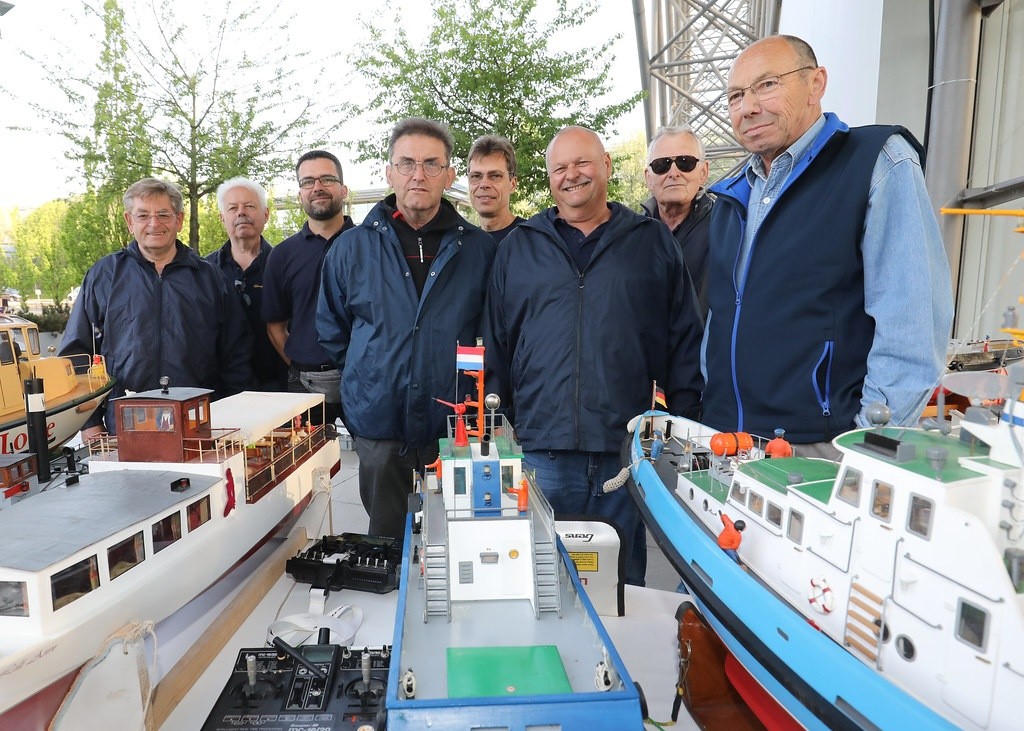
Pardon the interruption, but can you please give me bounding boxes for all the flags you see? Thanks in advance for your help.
[655,386,668,408]
[456,346,485,370]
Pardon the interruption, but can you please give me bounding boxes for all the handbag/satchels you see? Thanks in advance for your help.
[290,369,344,404]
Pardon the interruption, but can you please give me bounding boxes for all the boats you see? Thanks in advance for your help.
[379,337,650,731]
[0,311,119,465]
[0,375,341,731]
[614,386,1024,729]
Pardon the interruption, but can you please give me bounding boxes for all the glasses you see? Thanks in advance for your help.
[647,156,704,176]
[392,159,447,177]
[129,212,179,223]
[299,175,342,189]
[719,66,816,111]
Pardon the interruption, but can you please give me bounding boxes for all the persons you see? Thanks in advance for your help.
[261,150,357,425]
[718,514,746,563]
[765,428,792,459]
[505,480,528,515]
[425,452,442,494]
[57,177,260,447]
[314,118,498,536]
[0,332,22,361]
[651,430,664,467]
[482,125,705,616]
[699,35,955,462]
[304,421,315,434]
[467,135,528,241]
[983,335,991,353]
[641,125,714,295]
[203,176,292,391]
